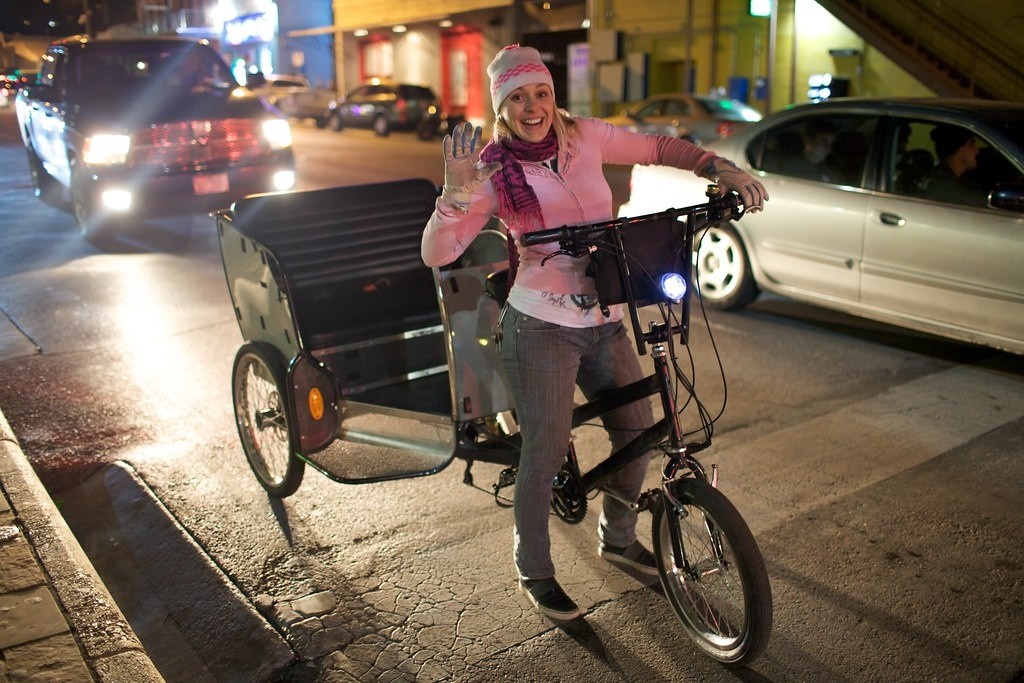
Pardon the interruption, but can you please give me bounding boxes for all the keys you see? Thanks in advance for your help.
[491,324,502,352]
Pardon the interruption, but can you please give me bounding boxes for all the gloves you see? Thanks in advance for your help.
[442,123,502,204]
[701,156,768,213]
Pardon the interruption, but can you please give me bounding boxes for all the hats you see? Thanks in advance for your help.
[487,43,555,116]
[929,125,975,161]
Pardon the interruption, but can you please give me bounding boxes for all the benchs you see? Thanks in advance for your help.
[217,178,478,355]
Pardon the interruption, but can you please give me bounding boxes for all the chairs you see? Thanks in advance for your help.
[898,149,935,198]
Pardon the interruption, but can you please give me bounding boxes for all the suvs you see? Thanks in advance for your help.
[15,35,296,245]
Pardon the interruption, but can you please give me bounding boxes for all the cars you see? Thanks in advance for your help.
[596,92,763,147]
[242,74,331,128]
[329,79,440,137]
[617,97,1024,357]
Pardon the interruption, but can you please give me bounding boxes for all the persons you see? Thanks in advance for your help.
[780,117,844,182]
[866,120,1001,209]
[421,44,769,622]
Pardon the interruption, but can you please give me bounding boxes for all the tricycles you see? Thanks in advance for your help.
[209,177,773,667]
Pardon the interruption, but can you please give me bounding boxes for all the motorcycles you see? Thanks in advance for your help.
[418,104,465,142]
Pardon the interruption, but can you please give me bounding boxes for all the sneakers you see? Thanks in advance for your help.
[598,540,659,576]
[518,576,579,620]
